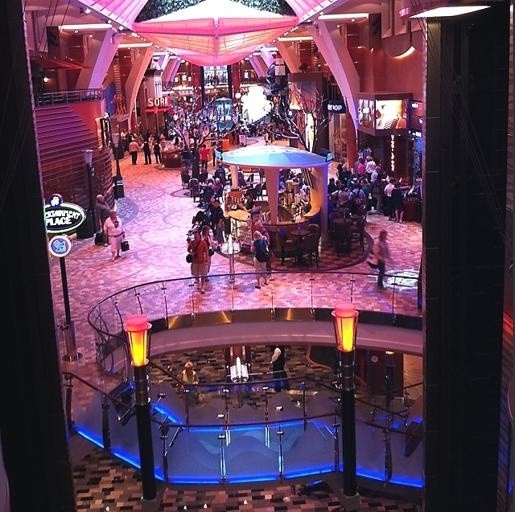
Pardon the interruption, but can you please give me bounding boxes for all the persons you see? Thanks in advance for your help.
[187,230,209,293]
[251,231,270,288]
[123,74,422,267]
[179,361,201,407]
[104,210,125,261]
[96,194,111,246]
[372,229,392,288]
[269,343,291,394]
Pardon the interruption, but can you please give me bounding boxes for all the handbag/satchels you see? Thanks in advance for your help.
[121,241,129,251]
[366,250,379,269]
[186,254,192,262]
[257,249,269,262]
[209,249,214,256]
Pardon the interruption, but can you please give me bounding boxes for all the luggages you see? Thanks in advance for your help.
[95,226,105,245]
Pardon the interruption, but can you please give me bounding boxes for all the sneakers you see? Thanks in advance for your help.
[255,282,268,288]
[111,256,115,262]
[197,276,209,293]
[103,243,108,247]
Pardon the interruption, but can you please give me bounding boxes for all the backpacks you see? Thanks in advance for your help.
[352,190,362,206]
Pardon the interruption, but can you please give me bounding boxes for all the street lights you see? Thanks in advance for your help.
[81,148,100,232]
[121,314,162,510]
[329,300,362,511]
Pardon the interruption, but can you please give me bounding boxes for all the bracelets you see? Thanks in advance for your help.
[270,361,272,365]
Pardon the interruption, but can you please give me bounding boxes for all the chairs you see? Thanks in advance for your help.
[180,171,367,266]
